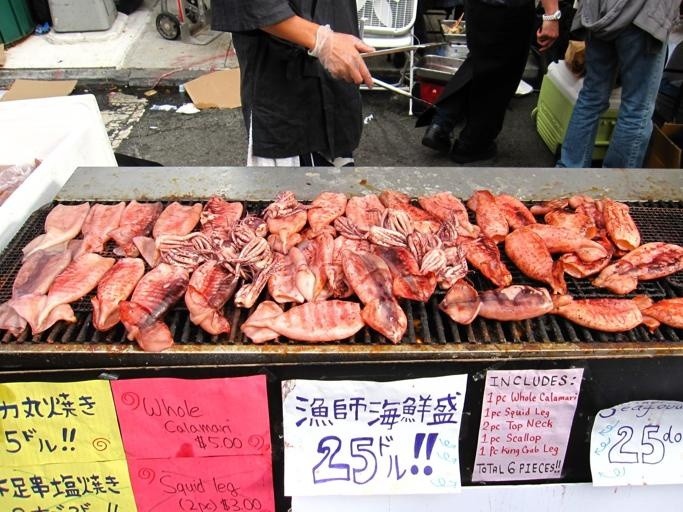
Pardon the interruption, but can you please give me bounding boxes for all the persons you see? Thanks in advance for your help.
[420,0,563,164]
[209,0,375,166]
[556,0,683,169]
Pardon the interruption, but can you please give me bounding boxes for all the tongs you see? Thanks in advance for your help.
[359,41,449,110]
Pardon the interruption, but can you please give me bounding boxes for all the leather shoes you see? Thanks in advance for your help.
[422,123,453,154]
[450,140,497,164]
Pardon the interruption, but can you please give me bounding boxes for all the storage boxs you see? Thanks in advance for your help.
[530,60,622,161]
[651,122,683,170]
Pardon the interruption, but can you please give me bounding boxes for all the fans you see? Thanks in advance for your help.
[356,1,418,37]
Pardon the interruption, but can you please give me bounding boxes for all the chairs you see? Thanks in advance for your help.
[357,29,414,116]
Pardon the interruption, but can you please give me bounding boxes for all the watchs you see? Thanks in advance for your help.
[542,9,562,21]
